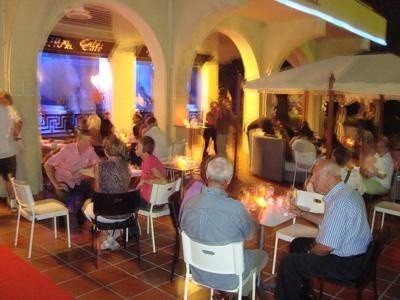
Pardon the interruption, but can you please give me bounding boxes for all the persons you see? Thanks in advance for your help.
[178,154,214,221]
[202,96,397,198]
[42,110,172,252]
[274,158,374,300]
[180,157,268,300]
[0,89,23,214]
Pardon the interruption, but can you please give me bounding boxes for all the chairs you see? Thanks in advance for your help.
[179,230,270,300]
[92,189,143,269]
[292,139,317,187]
[272,190,400,299]
[168,190,181,281]
[138,177,183,253]
[370,200,400,234]
[9,177,72,259]
[158,139,194,187]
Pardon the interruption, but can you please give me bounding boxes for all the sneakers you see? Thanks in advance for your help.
[241,295,250,300]
[214,290,227,299]
[100,240,110,250]
[107,236,120,250]
[10,200,17,209]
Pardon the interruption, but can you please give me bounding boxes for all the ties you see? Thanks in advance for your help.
[344,170,351,181]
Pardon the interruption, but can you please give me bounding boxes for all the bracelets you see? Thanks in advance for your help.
[300,211,304,218]
